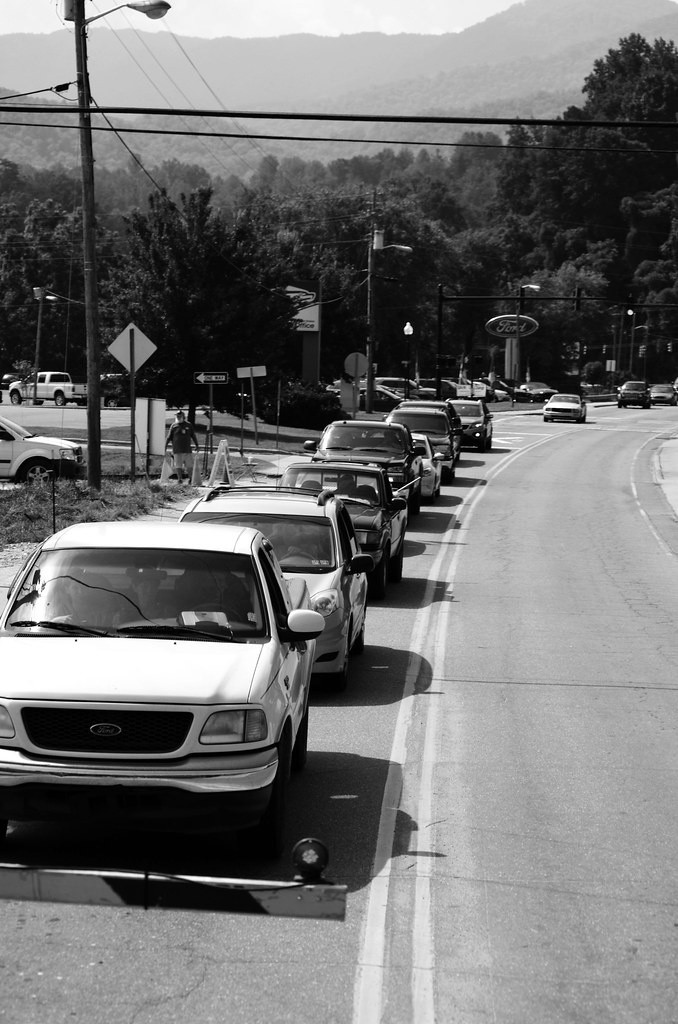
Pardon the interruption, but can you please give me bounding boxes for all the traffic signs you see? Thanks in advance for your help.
[193,371,229,385]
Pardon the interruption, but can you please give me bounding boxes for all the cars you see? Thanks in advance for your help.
[0,415,85,486]
[648,385,678,406]
[410,433,444,506]
[384,401,463,483]
[543,393,587,423]
[326,378,559,412]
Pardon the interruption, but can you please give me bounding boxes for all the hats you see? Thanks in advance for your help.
[175,411,184,417]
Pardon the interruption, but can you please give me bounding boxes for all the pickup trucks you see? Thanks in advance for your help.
[278,461,408,599]
[9,372,87,406]
[303,421,426,523]
[0,520,325,840]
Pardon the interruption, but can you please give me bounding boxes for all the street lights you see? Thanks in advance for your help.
[62,0,171,492]
[366,240,412,413]
[404,320,411,400]
[627,309,648,381]
[33,287,58,402]
[517,285,541,383]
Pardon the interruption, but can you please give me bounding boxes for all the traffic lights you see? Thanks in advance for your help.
[571,285,582,313]
[667,343,673,352]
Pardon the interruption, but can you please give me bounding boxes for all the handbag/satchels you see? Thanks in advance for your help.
[192,454,202,486]
[161,457,174,482]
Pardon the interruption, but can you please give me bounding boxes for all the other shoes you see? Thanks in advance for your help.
[177,479,181,484]
[188,479,192,484]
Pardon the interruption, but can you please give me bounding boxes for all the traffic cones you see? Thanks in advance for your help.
[219,464,229,484]
[190,455,205,487]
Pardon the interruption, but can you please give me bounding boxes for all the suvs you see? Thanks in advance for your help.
[445,398,493,452]
[616,381,651,410]
[179,485,375,696]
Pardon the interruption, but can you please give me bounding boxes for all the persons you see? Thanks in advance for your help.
[376,431,402,450]
[45,570,97,624]
[274,524,320,560]
[121,577,177,625]
[165,409,200,483]
